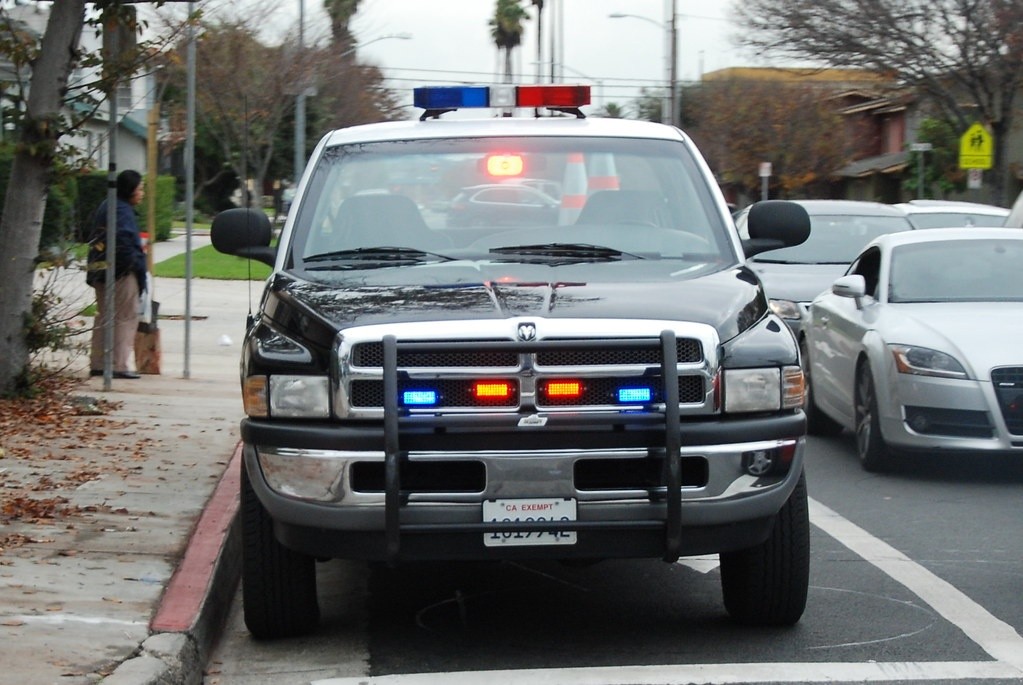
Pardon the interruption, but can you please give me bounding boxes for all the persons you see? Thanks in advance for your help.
[85,170,149,380]
[272,179,290,230]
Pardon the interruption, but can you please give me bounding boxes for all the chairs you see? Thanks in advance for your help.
[327,191,438,252]
[576,189,654,227]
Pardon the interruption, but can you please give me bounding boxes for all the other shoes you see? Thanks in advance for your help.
[90,369,102,375]
[113,371,140,378]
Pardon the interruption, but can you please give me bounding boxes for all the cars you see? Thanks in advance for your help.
[888,188,1023,231]
[794,225,1022,473]
[737,192,917,337]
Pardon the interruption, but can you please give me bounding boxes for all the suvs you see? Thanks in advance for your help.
[442,175,565,229]
[209,83,811,642]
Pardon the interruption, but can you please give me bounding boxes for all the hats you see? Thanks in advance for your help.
[117,170,142,197]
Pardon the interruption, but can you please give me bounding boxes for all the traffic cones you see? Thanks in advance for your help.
[584,152,620,194]
[556,149,588,225]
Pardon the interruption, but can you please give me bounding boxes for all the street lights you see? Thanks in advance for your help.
[291,31,412,190]
[530,58,607,117]
[609,12,681,126]
